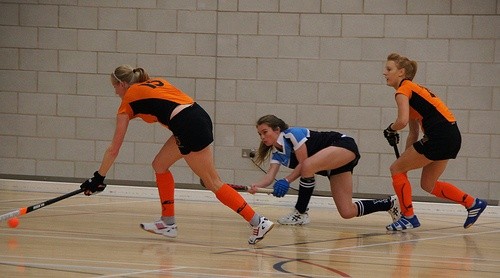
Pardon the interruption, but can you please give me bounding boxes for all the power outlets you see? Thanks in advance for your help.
[241,148,252,158]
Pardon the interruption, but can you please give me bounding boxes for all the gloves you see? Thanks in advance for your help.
[272,178,290,198]
[384,123,399,146]
[81,173,106,197]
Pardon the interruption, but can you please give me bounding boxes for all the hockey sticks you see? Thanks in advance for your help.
[390,132,399,158]
[200,177,275,193]
[0,188,85,222]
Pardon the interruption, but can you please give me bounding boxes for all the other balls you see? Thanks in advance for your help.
[7,217,18,227]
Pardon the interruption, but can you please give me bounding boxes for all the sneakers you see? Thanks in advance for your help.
[386,214,421,231]
[464,197,488,228]
[140,221,178,238]
[277,207,309,224]
[386,194,400,221]
[248,217,275,244]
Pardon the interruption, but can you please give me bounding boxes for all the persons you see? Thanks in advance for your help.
[80,65,275,245]
[246,115,403,226]
[382,54,488,232]
